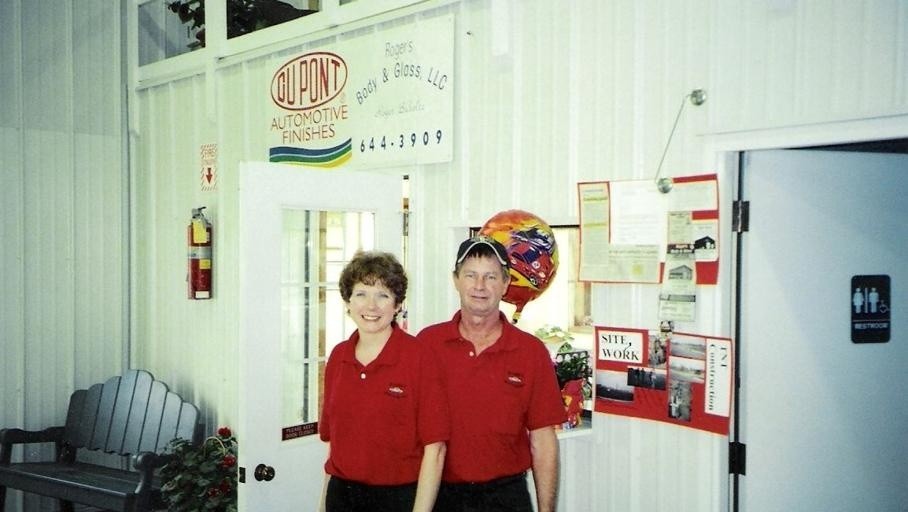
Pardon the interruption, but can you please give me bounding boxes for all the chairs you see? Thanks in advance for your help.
[0,370,200,512]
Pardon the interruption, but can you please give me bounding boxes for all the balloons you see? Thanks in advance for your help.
[476,209,561,323]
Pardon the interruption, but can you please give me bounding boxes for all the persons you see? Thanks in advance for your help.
[417,235,569,512]
[649,340,666,363]
[317,249,452,511]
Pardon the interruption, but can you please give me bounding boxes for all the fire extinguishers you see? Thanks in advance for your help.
[187,206,212,300]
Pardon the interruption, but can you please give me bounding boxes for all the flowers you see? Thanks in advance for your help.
[160,427,238,512]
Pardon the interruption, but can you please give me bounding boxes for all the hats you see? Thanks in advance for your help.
[456,236,511,269]
